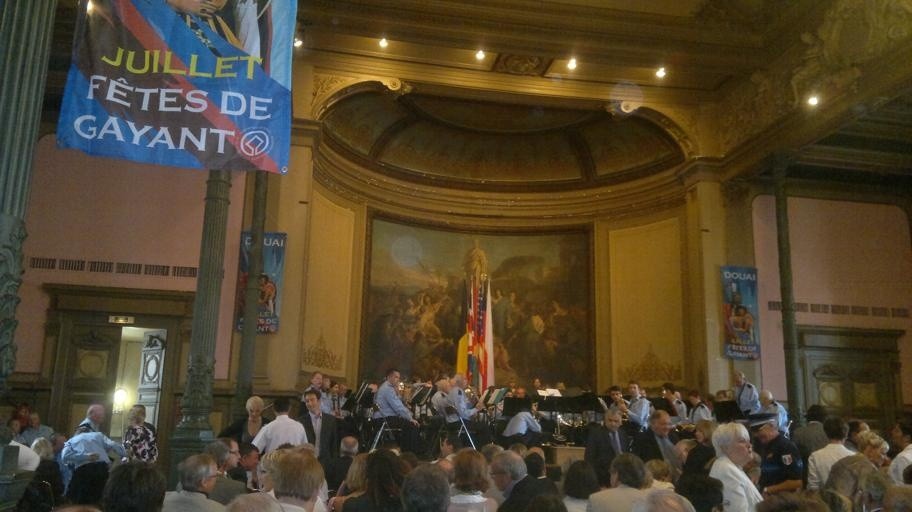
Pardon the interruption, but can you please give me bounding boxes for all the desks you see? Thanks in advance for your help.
[547,444,586,466]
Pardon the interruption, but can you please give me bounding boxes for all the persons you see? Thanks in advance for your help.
[729,305,753,344]
[1,366,912,511]
[88,0,246,153]
[388,289,584,384]
[724,291,742,337]
[260,276,276,316]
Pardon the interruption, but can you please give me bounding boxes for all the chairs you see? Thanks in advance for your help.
[30,480,54,508]
[368,404,403,452]
[434,406,479,450]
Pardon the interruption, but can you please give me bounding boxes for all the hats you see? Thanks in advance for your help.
[746,412,779,430]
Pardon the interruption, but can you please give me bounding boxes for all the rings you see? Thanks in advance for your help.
[200,9,205,14]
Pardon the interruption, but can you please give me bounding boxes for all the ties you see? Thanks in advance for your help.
[611,431,619,455]
